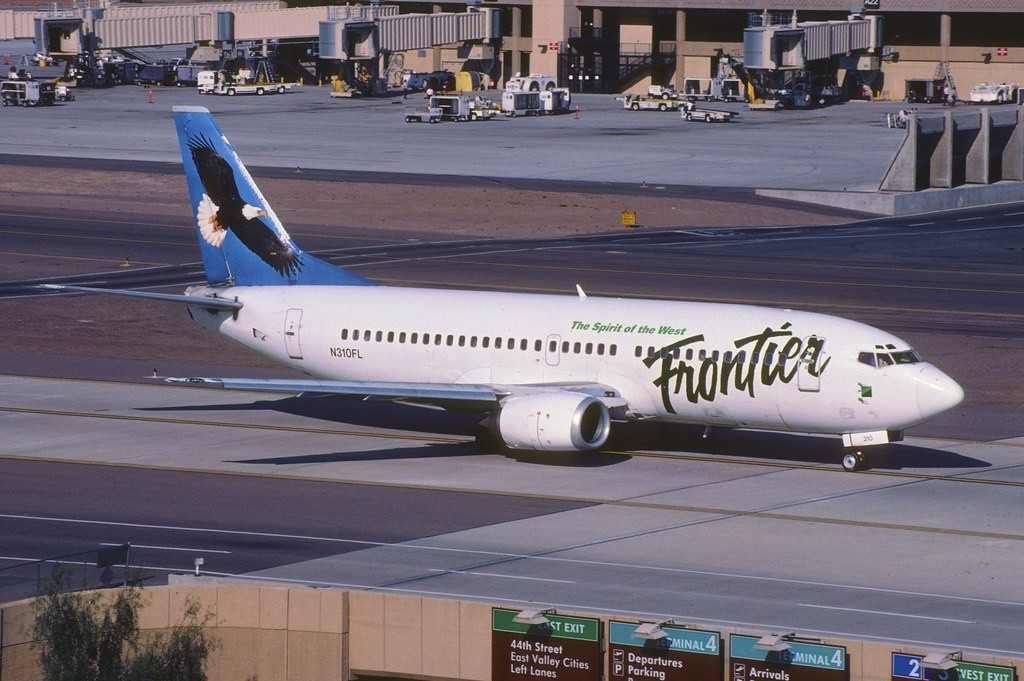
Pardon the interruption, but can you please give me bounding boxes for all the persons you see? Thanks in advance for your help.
[423,79,427,96]
[26,53,46,66]
[950,87,957,106]
[426,87,433,99]
[403,80,407,99]
[688,101,695,111]
[942,84,950,106]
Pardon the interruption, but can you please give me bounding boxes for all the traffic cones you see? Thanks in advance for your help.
[146,90,154,103]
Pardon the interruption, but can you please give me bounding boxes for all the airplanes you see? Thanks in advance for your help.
[13,103,968,475]
